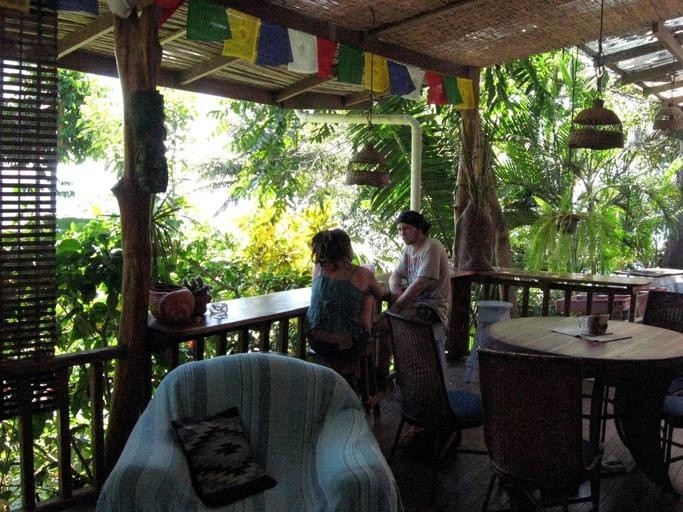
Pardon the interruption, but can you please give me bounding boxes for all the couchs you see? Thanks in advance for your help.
[94,350,402,512]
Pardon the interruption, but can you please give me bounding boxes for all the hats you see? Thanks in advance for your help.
[394,211,430,234]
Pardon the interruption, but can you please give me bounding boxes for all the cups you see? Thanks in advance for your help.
[210,302,228,315]
[578,314,610,334]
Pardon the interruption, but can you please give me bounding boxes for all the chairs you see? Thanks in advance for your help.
[600,292,681,476]
[475,346,604,510]
[386,298,488,499]
[346,4,392,188]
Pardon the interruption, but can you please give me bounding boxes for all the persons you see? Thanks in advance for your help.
[302,228,386,359]
[374,209,454,396]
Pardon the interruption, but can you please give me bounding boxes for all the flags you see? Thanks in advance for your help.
[425,71,475,110]
[316,37,336,79]
[403,67,425,101]
[186,3,232,40]
[287,28,318,73]
[364,53,390,93]
[223,8,261,63]
[338,45,363,84]
[257,22,293,65]
[158,3,182,26]
[49,1,99,15]
[387,60,416,95]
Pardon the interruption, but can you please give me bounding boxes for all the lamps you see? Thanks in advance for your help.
[567,49,625,151]
[651,58,683,132]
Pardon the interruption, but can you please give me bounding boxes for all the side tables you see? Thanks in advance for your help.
[488,316,682,510]
